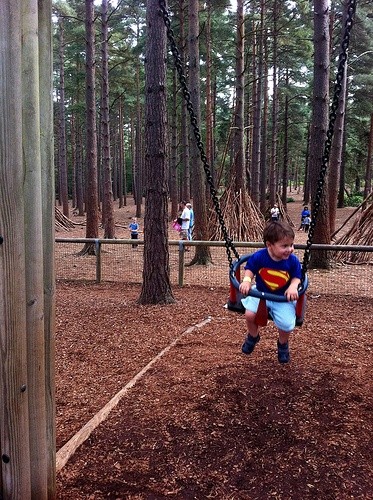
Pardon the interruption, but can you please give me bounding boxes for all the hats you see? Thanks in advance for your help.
[186,203,192,208]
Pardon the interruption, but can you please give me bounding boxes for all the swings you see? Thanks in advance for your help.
[157,1,357,326]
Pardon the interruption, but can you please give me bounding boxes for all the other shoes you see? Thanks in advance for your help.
[278,340,289,362]
[242,333,260,355]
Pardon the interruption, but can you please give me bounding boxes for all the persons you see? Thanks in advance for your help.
[173,201,195,251]
[271,204,281,222]
[297,206,312,233]
[239,222,302,364]
[128,217,139,248]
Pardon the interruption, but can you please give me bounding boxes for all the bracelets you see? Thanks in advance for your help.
[243,276,252,283]
